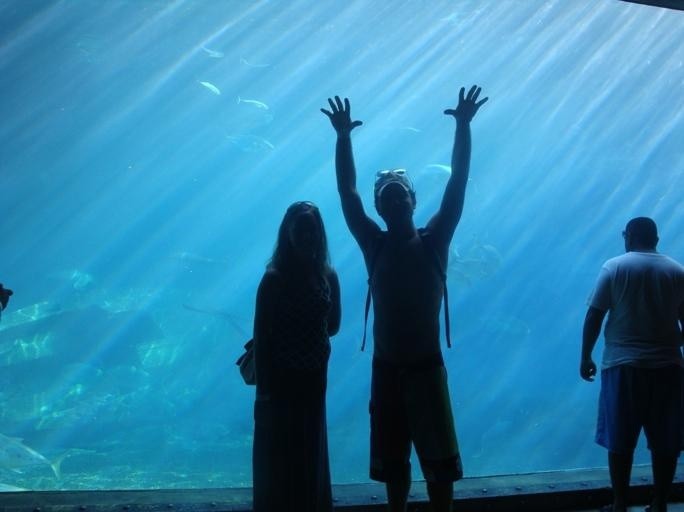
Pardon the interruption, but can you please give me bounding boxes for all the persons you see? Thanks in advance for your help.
[582,217,684,509]
[235,200,342,510]
[320,82,489,511]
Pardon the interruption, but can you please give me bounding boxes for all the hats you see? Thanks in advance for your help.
[373,173,412,197]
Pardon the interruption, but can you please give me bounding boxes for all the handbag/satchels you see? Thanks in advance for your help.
[235,336,256,387]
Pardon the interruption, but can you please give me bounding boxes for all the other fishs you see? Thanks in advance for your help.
[172,251,221,269]
[237,96,269,114]
[224,133,274,154]
[43,266,98,287]
[201,45,226,59]
[448,243,511,289]
[0,284,231,493]
[424,164,472,184]
[196,79,221,97]
[241,59,273,75]
